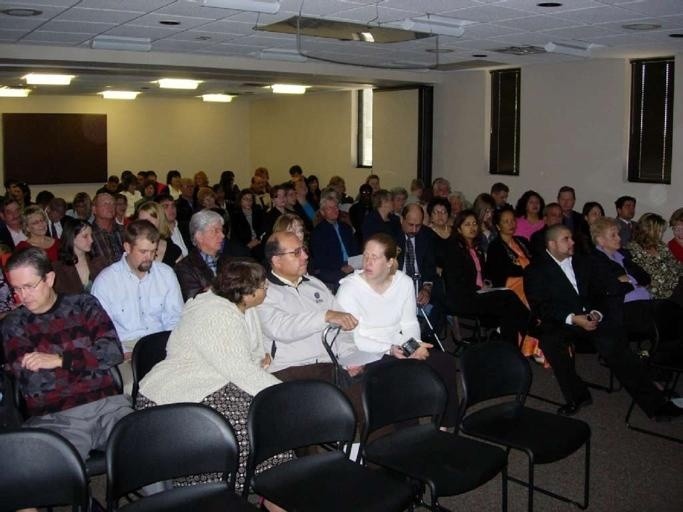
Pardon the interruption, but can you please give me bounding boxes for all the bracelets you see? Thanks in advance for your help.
[54,350,64,364]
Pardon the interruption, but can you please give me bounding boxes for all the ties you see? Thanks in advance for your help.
[406,237,415,276]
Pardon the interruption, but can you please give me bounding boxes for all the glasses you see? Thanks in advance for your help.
[277,245,307,257]
[15,279,41,293]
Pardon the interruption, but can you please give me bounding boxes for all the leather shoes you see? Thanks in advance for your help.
[558,397,592,416]
[656,401,682,421]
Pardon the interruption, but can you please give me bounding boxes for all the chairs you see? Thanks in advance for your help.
[0,270,683,512]
[242,378,424,512]
[130,326,173,408]
[319,271,682,444]
[103,402,266,512]
[0,425,95,511]
[1,324,126,480]
[452,338,599,511]
[356,357,511,511]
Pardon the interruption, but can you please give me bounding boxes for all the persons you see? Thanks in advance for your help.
[523,224,681,425]
[2,245,154,511]
[86,219,185,396]
[0,163,682,341]
[242,229,366,461]
[132,258,296,510]
[587,216,682,402]
[332,232,434,383]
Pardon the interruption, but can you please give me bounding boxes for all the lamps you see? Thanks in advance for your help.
[400,17,464,39]
[255,50,307,63]
[392,62,429,72]
[198,0,284,16]
[80,35,152,52]
[544,41,591,59]
